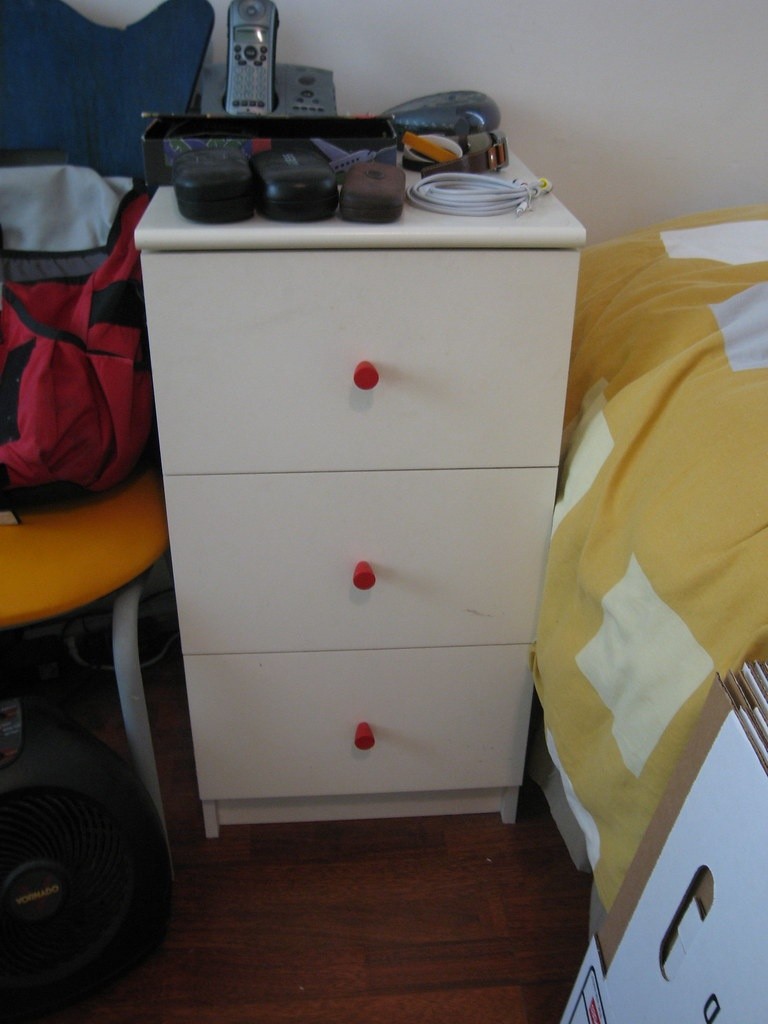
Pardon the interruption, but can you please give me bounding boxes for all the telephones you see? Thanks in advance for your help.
[198,0,338,116]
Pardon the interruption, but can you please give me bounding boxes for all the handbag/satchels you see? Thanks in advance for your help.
[0,191,146,509]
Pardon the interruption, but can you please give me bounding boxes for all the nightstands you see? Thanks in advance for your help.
[133,152,586,841]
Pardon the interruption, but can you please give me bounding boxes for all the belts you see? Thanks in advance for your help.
[399,133,508,179]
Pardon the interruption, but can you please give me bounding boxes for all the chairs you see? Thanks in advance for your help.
[0,0,217,879]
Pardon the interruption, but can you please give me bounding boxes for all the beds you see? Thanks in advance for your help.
[529,202,768,952]
